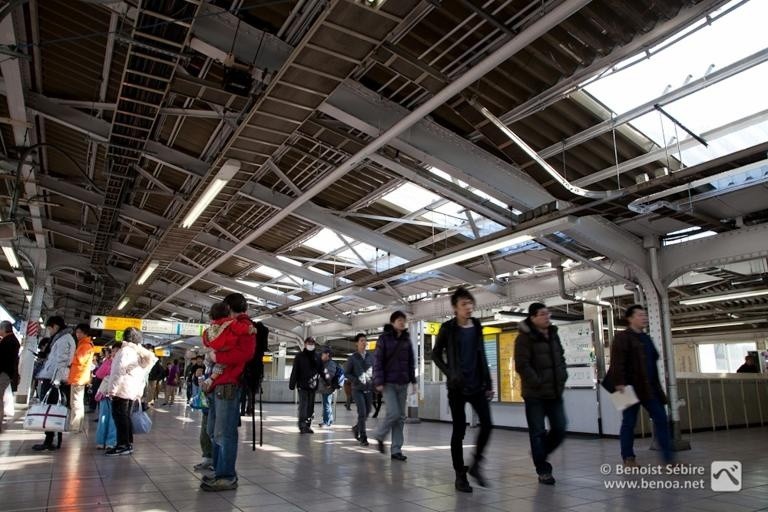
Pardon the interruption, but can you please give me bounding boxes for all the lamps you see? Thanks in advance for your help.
[0,241,33,303]
[38,316,46,329]
[135,260,159,285]
[405,203,580,276]
[170,334,184,344]
[179,159,241,229]
[287,252,362,312]
[117,297,130,311]
[0,304,16,325]
[676,258,768,306]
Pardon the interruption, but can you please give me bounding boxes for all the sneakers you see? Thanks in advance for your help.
[671,459,687,467]
[392,453,407,461]
[623,461,641,469]
[360,440,369,446]
[193,462,238,492]
[352,427,358,440]
[378,440,384,454]
[538,474,555,485]
[468,469,483,485]
[96,442,132,456]
[199,381,208,393]
[32,442,61,451]
[455,481,473,491]
[300,428,313,434]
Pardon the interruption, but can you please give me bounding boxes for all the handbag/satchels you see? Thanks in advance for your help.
[131,411,152,433]
[331,367,344,389]
[24,402,71,431]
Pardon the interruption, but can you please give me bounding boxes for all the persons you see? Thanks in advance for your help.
[0,294,384,490]
[513,301,568,484]
[430,288,495,493]
[736,356,760,373]
[371,310,417,461]
[601,304,686,471]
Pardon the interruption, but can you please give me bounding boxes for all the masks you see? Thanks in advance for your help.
[307,344,315,350]
